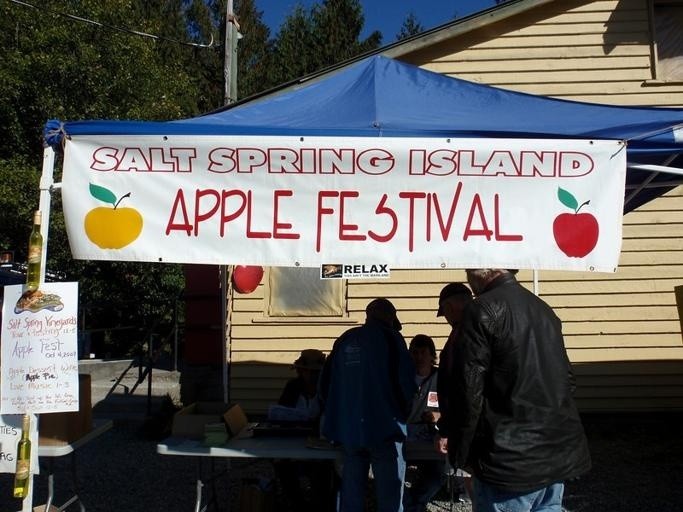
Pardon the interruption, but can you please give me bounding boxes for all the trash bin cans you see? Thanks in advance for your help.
[93,393,175,469]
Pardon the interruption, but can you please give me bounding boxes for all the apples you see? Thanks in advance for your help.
[232,265,263,294]
[85,184,144,250]
[553,187,600,258]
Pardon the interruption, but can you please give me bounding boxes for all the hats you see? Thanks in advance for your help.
[294,349,324,370]
[366,299,402,331]
[437,283,472,316]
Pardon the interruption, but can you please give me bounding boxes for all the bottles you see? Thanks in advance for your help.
[25,209,43,291]
[11,414,31,498]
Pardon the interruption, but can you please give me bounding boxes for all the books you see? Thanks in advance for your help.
[266,403,313,424]
[301,432,335,450]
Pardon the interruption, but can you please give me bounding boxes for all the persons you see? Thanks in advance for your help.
[316,298,420,511]
[404,335,447,512]
[264,346,337,511]
[443,267,594,511]
[433,282,475,456]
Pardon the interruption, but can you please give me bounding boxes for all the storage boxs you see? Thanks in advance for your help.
[172,400,247,438]
[40,374,92,446]
[239,483,264,512]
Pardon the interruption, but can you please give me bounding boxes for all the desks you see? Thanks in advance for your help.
[39,414,113,512]
[157,425,452,512]
